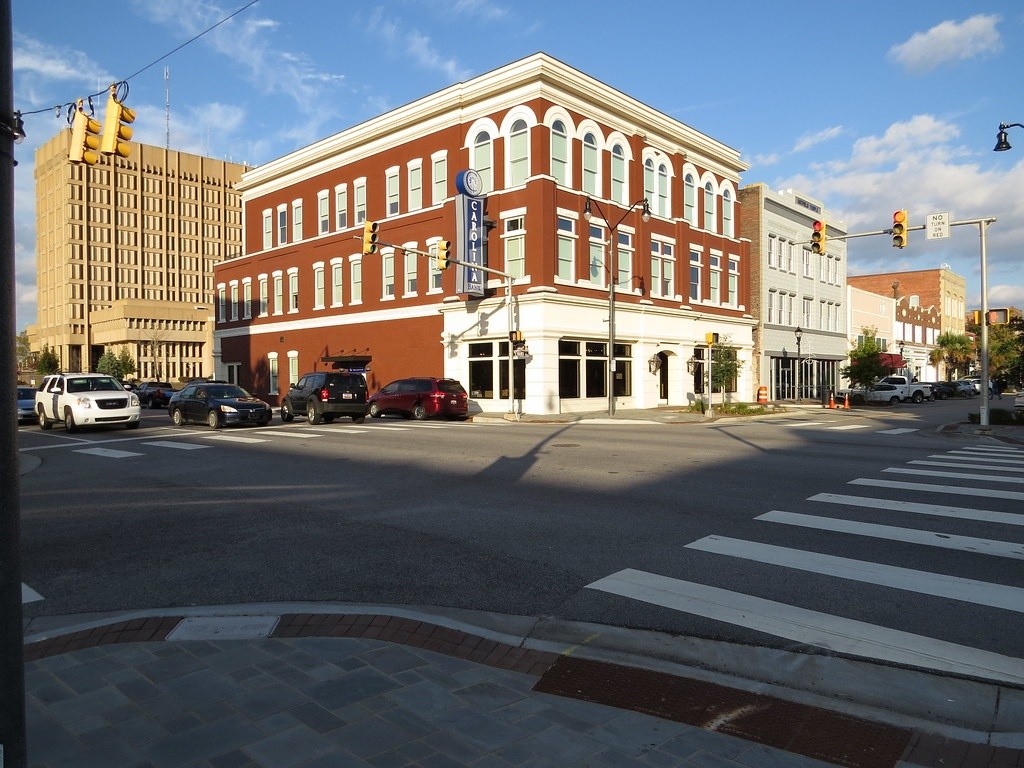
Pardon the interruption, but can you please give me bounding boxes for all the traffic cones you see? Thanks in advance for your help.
[842,392,852,409]
[827,392,836,409]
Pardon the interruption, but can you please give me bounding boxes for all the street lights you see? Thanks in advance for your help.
[794,326,804,402]
[899,340,905,376]
[582,195,653,416]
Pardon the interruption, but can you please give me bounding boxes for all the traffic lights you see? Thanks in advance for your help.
[68,111,102,164]
[892,210,907,250]
[101,98,137,157]
[509,330,521,342]
[438,240,451,271]
[363,220,380,256]
[811,218,826,256]
[988,308,1010,326]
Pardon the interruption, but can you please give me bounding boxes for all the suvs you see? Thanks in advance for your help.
[34,372,141,434]
[281,371,370,425]
[130,382,174,409]
[835,381,905,408]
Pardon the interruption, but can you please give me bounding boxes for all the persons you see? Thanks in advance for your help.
[911,376,918,383]
[989,379,1002,400]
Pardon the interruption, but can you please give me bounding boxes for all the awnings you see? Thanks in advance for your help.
[879,353,907,368]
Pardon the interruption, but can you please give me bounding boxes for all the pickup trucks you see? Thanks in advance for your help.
[870,375,933,404]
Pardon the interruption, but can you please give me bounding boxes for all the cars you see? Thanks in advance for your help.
[16,385,40,426]
[909,375,995,401]
[368,376,469,421]
[168,381,273,429]
